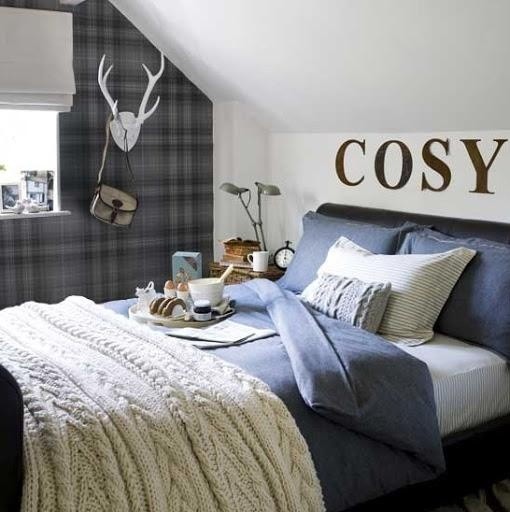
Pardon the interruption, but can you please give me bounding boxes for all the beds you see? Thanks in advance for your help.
[0,202,510,512]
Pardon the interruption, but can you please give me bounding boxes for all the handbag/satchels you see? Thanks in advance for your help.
[87,183,137,230]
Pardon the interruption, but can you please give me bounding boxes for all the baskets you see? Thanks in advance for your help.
[225,239,260,256]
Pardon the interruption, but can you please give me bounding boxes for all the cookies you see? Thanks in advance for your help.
[149,296,186,317]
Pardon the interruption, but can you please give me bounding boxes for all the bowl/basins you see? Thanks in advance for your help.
[188,278,225,306]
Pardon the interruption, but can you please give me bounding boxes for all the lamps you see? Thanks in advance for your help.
[218,181,282,265]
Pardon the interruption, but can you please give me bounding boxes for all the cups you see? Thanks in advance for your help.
[163,288,189,301]
[247,251,270,273]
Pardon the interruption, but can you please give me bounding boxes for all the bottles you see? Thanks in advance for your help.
[192,299,213,322]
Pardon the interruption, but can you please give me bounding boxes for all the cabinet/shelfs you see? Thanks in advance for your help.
[207,262,284,286]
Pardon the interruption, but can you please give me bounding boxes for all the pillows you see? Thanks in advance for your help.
[275,209,510,367]
